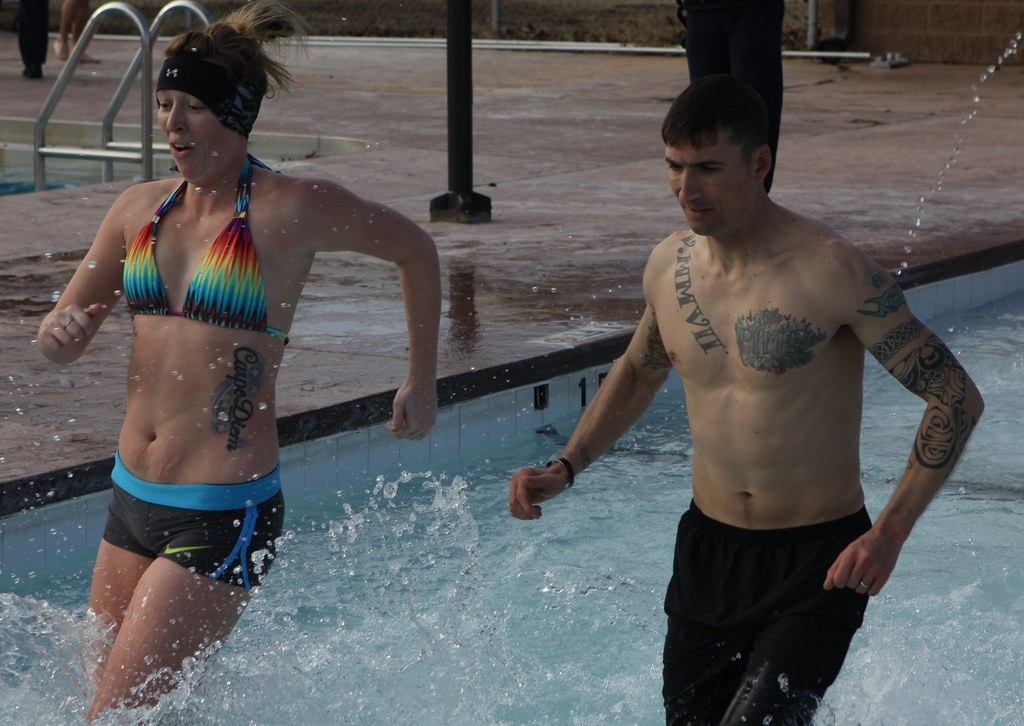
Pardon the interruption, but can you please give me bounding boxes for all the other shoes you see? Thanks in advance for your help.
[23,65,41,78]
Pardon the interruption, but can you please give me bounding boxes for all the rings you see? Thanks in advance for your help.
[860,580,870,588]
[64,319,73,329]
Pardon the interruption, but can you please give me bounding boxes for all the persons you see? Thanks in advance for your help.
[508,74,984,726]
[54,0,90,59]
[19,0,48,78]
[679,0,785,194]
[36,0,441,726]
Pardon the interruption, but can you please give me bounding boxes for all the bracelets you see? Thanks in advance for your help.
[546,458,574,489]
